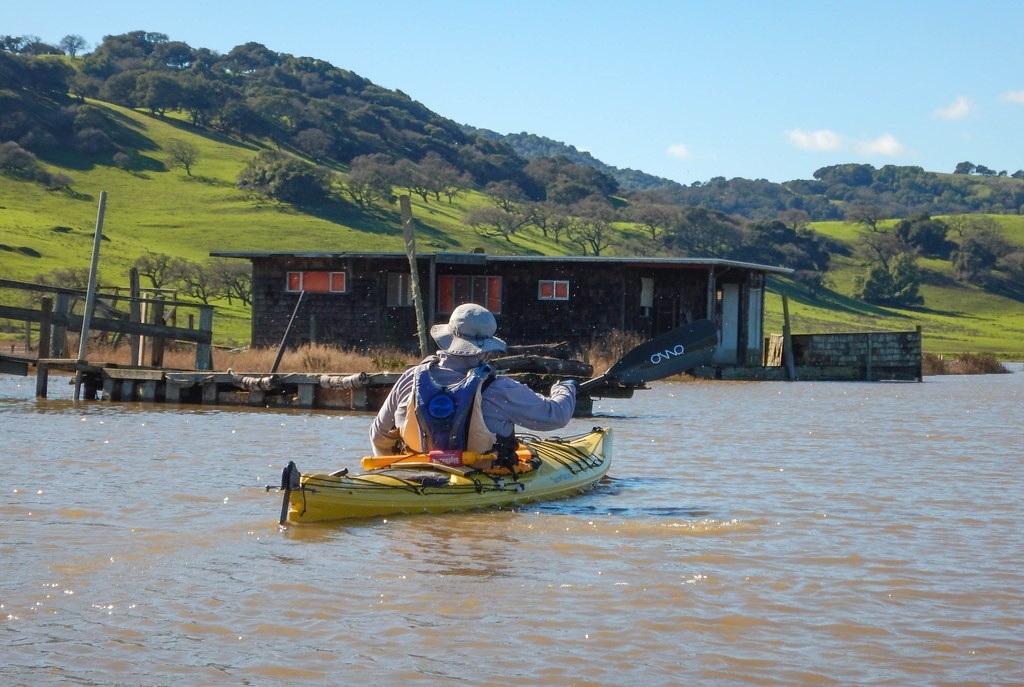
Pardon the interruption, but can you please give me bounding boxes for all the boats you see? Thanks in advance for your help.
[266,427,614,528]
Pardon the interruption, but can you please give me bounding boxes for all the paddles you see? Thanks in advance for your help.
[575,320,720,397]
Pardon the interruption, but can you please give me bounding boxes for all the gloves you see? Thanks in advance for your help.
[561,379,581,395]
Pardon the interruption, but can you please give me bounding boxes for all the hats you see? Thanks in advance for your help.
[430,303,508,356]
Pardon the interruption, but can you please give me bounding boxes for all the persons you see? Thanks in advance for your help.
[369,303,579,456]
